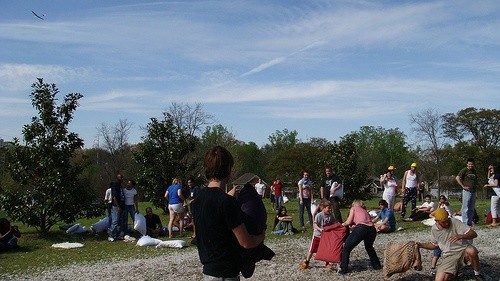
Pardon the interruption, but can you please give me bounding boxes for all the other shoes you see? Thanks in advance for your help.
[124,236,132,240]
[472,274,484,281]
[325,264,332,268]
[402,217,413,221]
[108,237,115,241]
[431,269,436,275]
[300,263,308,269]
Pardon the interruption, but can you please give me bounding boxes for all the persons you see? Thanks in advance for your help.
[270,177,284,212]
[339,200,381,271]
[371,200,396,233]
[296,170,313,228]
[101,160,197,243]
[255,178,266,200]
[189,146,260,281]
[413,157,500,281]
[401,163,418,217]
[299,200,335,269]
[380,165,403,212]
[320,167,343,223]
[0,218,21,253]
[272,206,294,235]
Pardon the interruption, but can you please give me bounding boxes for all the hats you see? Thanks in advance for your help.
[411,163,417,167]
[388,166,394,170]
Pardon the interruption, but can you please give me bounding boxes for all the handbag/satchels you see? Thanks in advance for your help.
[486,210,499,224]
[283,196,289,203]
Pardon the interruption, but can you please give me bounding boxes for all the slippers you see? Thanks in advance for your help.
[489,225,496,227]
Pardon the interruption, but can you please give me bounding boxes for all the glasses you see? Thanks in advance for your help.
[440,197,444,199]
